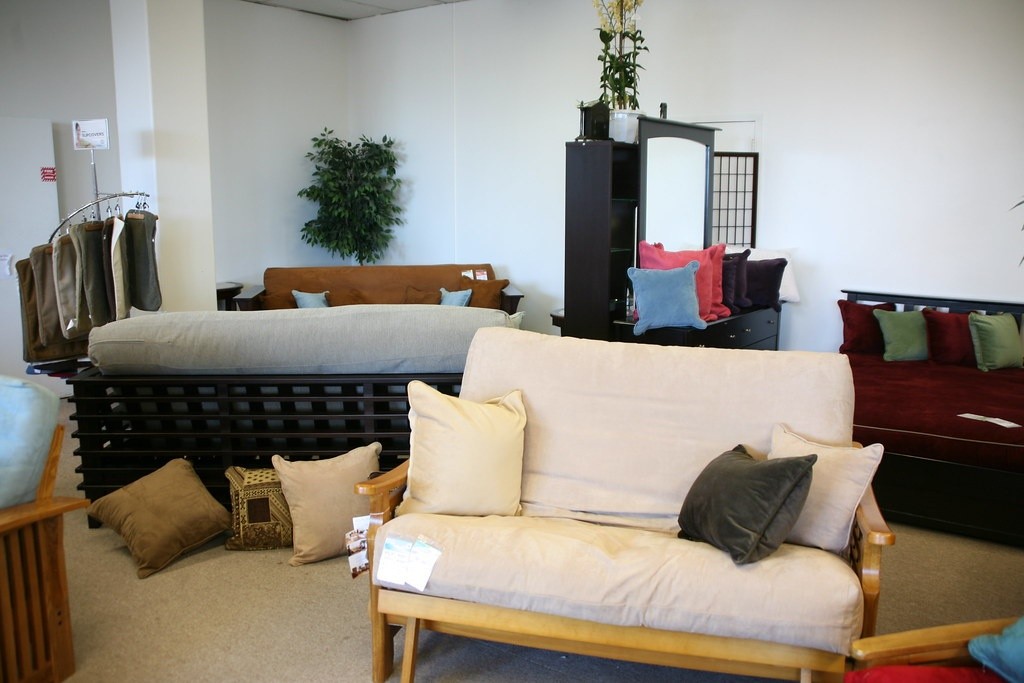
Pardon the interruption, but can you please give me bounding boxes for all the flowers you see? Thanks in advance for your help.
[589,0,650,110]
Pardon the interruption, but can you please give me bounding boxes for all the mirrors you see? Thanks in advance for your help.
[636,115,723,269]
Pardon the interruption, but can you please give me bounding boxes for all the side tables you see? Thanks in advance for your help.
[216,281,244,311]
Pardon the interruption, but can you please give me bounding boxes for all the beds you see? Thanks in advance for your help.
[842,289,1024,549]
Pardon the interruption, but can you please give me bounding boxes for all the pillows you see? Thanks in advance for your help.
[767,422,884,553]
[224,466,294,551]
[271,442,383,567]
[921,307,978,369]
[967,617,1024,683]
[258,292,297,310]
[325,289,365,307]
[290,289,330,308]
[676,444,818,565]
[968,311,1024,372]
[639,240,731,321]
[626,260,707,335]
[872,308,934,361]
[713,243,800,312]
[838,300,896,354]
[460,275,510,310]
[85,458,232,579]
[404,285,442,304]
[439,287,473,307]
[394,381,527,516]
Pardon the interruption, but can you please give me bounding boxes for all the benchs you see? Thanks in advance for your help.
[0,374,91,683]
[359,325,897,683]
[231,263,524,314]
[67,304,527,529]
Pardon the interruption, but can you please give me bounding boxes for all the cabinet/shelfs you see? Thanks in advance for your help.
[549,140,787,351]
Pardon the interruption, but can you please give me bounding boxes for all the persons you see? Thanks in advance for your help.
[76,123,95,148]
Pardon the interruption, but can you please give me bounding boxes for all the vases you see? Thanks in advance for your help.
[609,111,646,144]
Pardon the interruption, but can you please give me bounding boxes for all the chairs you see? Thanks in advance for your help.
[844,617,1024,683]
